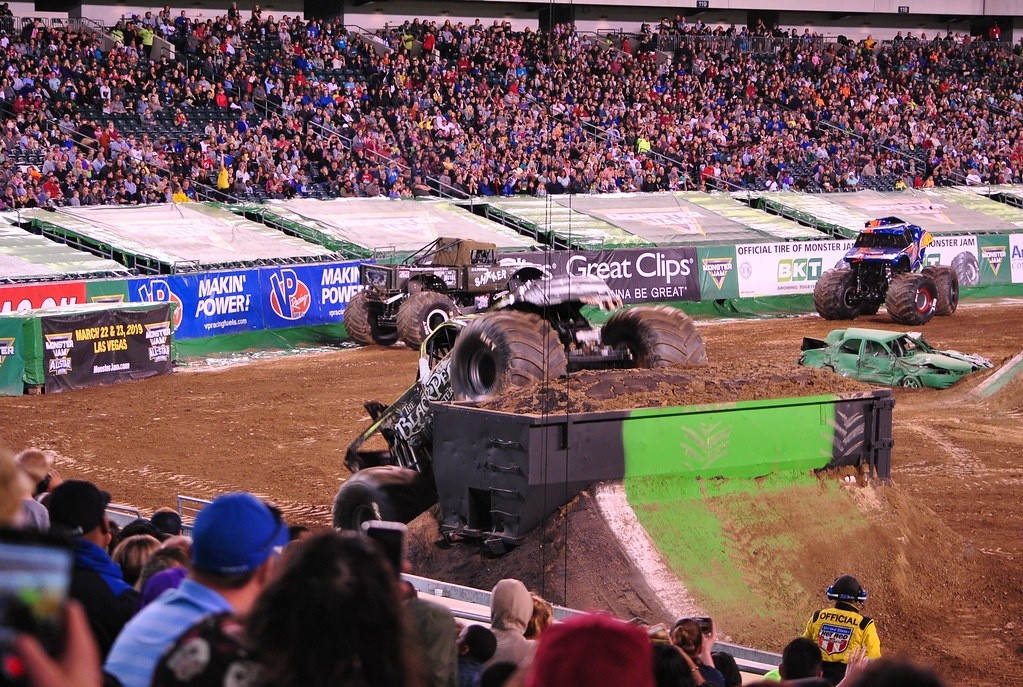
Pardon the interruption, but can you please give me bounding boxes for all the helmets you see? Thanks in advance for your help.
[826,575,867,603]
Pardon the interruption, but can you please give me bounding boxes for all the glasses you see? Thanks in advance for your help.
[262,499,283,547]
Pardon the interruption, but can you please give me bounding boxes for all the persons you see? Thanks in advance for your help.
[0,2,1023,212]
[801,575,882,664]
[0,447,952,687]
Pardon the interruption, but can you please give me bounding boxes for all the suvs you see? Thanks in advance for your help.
[330,264,707,536]
[811,216,959,325]
[344,240,552,350]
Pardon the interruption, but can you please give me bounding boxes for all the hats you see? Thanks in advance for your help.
[192,492,289,576]
[527,612,654,687]
[48,479,112,538]
[151,512,183,533]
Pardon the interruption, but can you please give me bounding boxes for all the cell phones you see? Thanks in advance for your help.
[648,623,672,644]
[0,523,77,687]
[691,617,712,634]
[364,521,405,582]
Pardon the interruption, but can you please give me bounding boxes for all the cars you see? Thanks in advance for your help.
[798,328,995,390]
